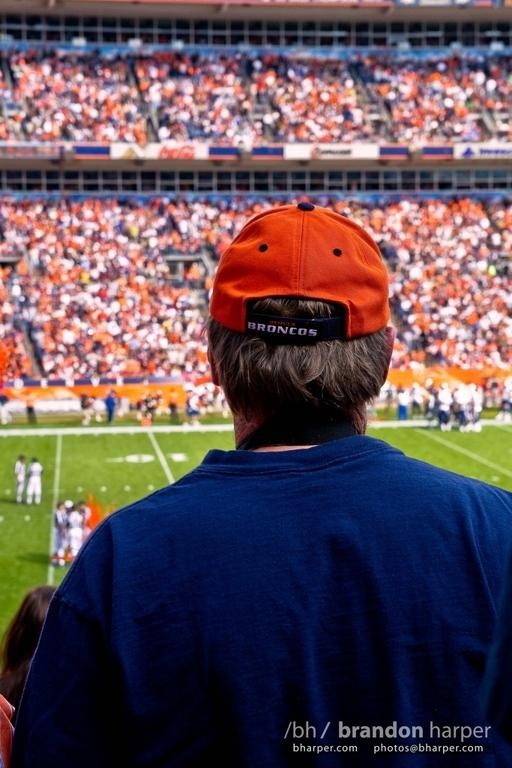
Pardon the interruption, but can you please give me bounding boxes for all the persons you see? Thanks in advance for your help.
[1,16,512,572]
[1,583,61,730]
[7,200,512,768]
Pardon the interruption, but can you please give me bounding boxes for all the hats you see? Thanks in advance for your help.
[209,202,391,342]
